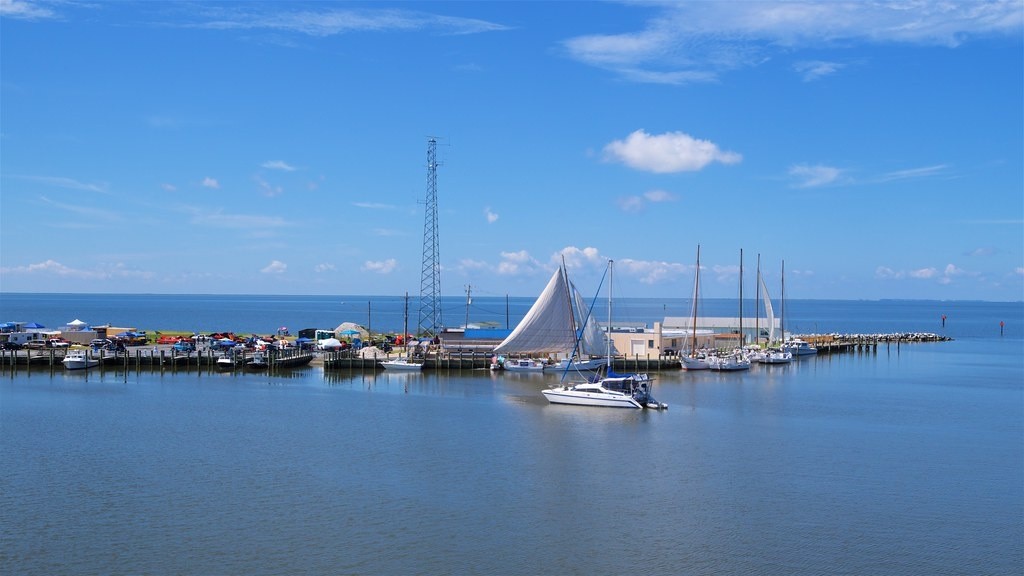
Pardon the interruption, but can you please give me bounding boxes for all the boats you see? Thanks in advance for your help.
[247,348,268,369]
[216,351,238,368]
[60,350,99,370]
[274,347,314,367]
[381,360,423,372]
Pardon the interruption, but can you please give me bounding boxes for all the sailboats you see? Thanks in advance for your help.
[543,260,669,410]
[677,243,818,372]
[490,253,618,372]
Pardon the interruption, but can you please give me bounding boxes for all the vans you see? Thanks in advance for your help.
[90,339,112,348]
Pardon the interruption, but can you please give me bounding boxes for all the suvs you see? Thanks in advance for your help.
[22,340,46,350]
[48,335,72,348]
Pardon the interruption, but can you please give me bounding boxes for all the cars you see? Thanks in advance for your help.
[155,330,279,353]
[0,341,22,351]
